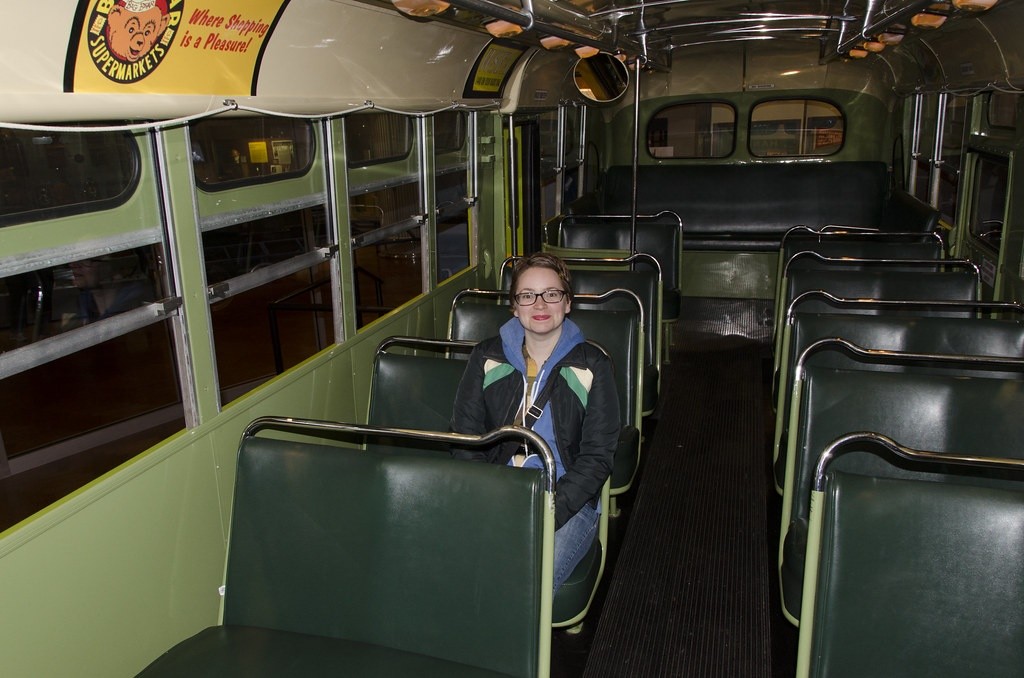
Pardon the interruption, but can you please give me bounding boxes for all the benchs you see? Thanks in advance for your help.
[134,414,558,678]
[372,159,1023,628]
[796,429,1024,678]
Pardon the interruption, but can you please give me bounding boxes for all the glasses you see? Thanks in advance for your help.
[513,288,569,306]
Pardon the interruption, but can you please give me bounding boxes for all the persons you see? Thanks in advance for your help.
[448,253,621,603]
